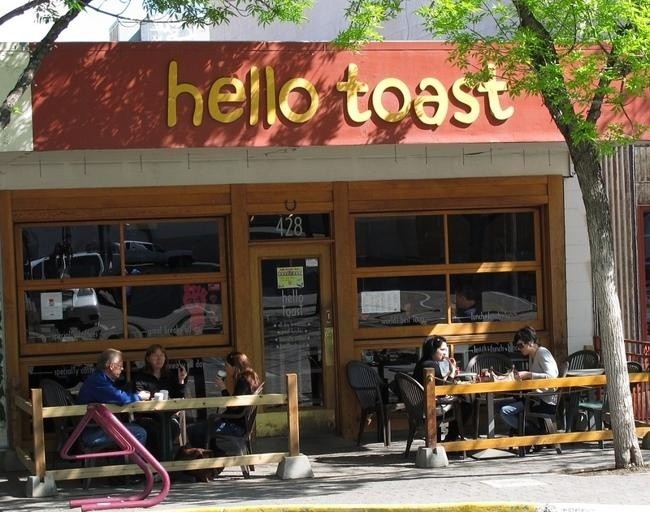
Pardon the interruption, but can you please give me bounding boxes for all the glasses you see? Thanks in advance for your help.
[516,342,525,349]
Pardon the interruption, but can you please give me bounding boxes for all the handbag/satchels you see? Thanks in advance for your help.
[174,447,224,481]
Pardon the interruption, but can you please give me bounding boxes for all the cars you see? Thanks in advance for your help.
[25,239,223,333]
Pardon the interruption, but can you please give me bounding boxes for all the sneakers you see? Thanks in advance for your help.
[447,451,463,460]
[525,445,545,454]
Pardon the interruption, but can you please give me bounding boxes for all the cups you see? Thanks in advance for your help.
[216,370,227,388]
[154,390,169,400]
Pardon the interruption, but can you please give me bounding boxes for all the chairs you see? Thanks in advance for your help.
[36,370,266,492]
[345,349,642,463]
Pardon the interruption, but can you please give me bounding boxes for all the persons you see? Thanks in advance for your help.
[75,347,152,490]
[453,283,481,320]
[183,350,260,473]
[409,334,472,460]
[132,343,190,482]
[498,323,560,454]
